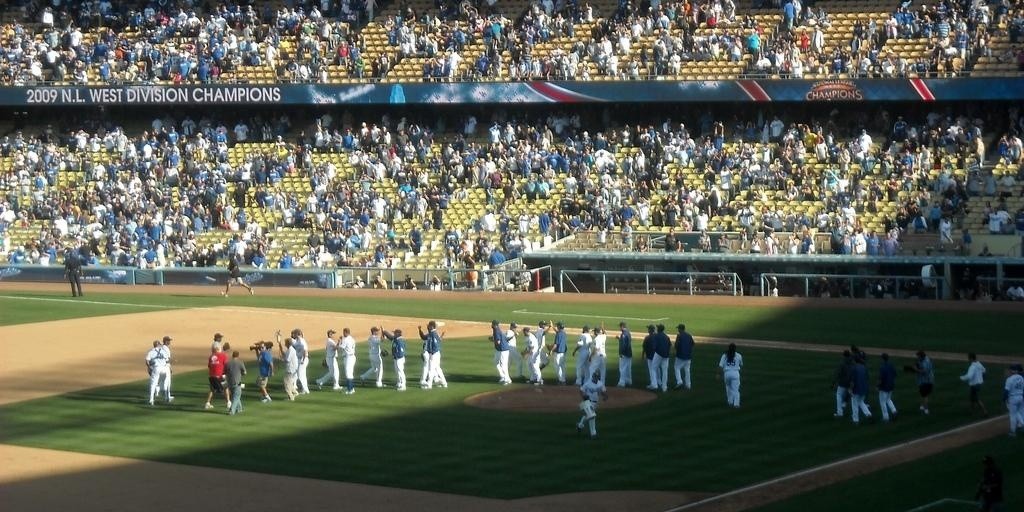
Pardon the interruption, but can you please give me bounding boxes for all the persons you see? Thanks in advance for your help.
[847,350,873,424]
[911,348,936,414]
[959,352,990,418]
[1001,361,1024,440]
[874,352,897,422]
[577,370,608,440]
[845,342,860,399]
[830,347,853,417]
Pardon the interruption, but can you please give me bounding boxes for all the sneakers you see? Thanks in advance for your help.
[589,434,600,440]
[1005,432,1017,441]
[220,291,229,298]
[576,423,585,434]
[722,402,990,427]
[249,287,254,297]
[492,369,694,394]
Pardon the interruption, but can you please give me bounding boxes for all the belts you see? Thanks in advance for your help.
[590,399,598,403]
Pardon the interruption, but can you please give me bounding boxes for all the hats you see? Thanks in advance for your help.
[392,329,402,333]
[834,343,926,361]
[488,319,689,335]
[285,328,303,348]
[163,336,172,342]
[427,321,436,327]
[214,333,223,338]
[153,341,162,348]
[371,327,380,332]
[327,330,336,336]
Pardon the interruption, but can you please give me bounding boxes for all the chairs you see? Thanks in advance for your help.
[0,0,1024,301]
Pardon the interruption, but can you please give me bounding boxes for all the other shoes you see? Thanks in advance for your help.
[316,379,323,390]
[155,392,159,397]
[397,386,406,391]
[332,384,343,390]
[345,388,356,394]
[360,376,365,386]
[224,401,244,415]
[165,396,174,403]
[284,386,309,401]
[419,381,448,389]
[396,383,401,388]
[376,385,387,388]
[148,399,154,405]
[204,403,214,409]
[260,397,272,403]
